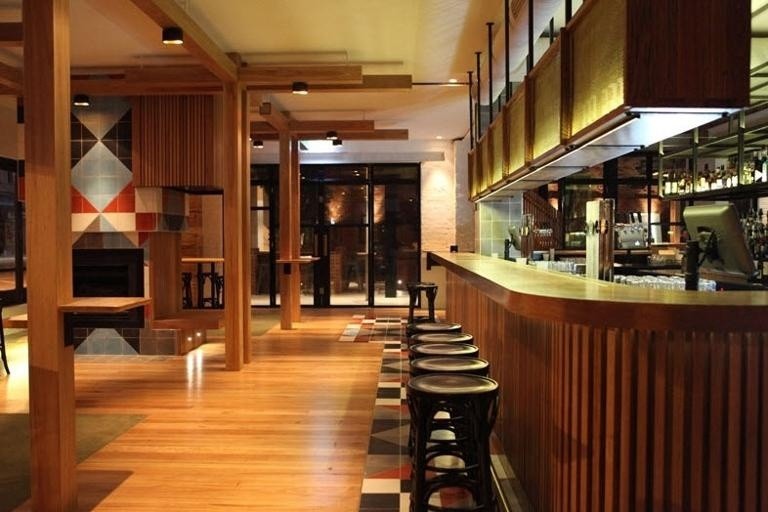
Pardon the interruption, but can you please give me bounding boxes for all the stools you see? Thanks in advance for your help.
[404,279,501,511]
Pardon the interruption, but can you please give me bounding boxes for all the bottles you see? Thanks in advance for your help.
[659,148,768,195]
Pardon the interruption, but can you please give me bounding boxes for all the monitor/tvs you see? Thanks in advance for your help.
[682,203,756,276]
[508,225,522,250]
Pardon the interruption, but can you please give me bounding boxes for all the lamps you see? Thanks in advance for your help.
[162,27,184,46]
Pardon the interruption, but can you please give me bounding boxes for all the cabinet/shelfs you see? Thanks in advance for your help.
[658,103,768,202]
